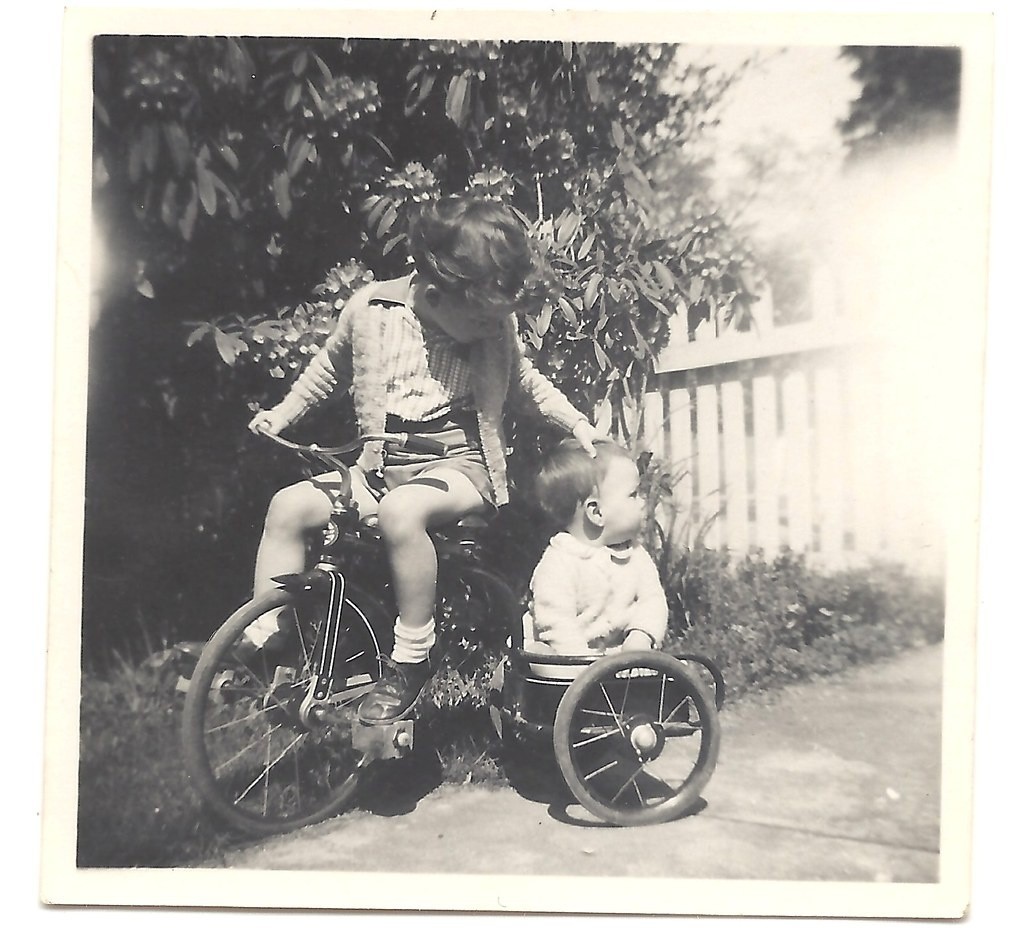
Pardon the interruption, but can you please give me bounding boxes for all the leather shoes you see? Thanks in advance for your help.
[357,645,446,725]
[223,650,287,700]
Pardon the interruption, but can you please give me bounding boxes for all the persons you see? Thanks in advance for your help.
[173,195,616,724]
[505,439,669,678]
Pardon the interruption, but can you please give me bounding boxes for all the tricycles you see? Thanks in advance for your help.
[179,421,725,834]
